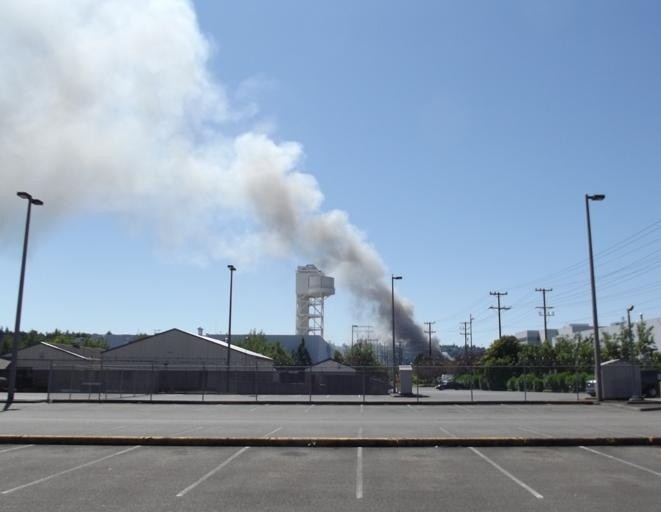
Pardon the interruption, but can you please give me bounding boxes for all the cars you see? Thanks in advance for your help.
[436,380,463,391]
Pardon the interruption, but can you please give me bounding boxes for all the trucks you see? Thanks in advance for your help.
[584,371,661,397]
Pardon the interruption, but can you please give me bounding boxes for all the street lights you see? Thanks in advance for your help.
[625,304,637,400]
[391,274,407,396]
[226,264,238,392]
[7,190,44,400]
[351,324,360,351]
[585,191,609,401]
